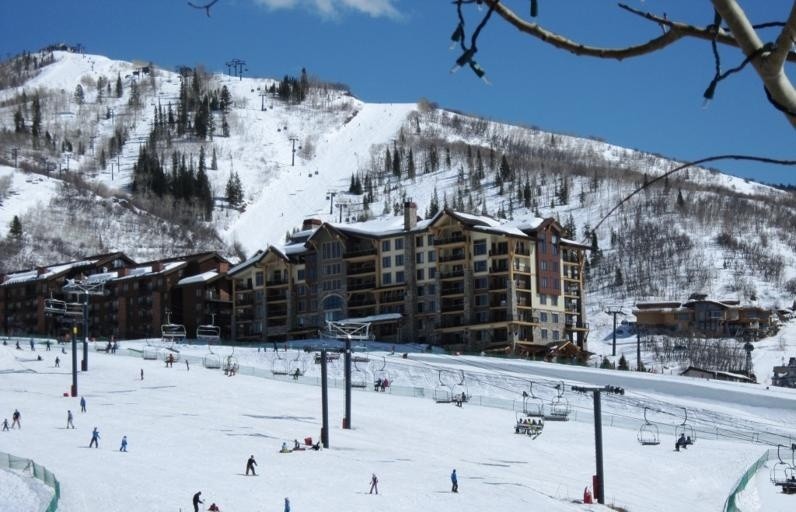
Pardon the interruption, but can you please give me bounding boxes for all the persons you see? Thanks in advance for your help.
[673,432,686,451]
[294,439,300,450]
[119,435,127,452]
[14,332,72,368]
[228,363,237,376]
[166,353,173,367]
[208,502,219,512]
[1,418,10,431]
[683,435,691,447]
[89,426,101,448]
[66,409,74,428]
[456,393,461,405]
[374,378,381,391]
[245,455,257,475]
[141,368,143,379]
[224,362,231,374]
[293,368,300,380]
[80,395,87,412]
[450,468,458,492]
[105,340,111,353]
[281,442,287,452]
[192,490,203,512]
[283,496,290,511]
[12,408,21,427]
[458,391,466,406]
[515,417,544,435]
[381,378,388,392]
[369,472,378,494]
[110,340,117,354]
